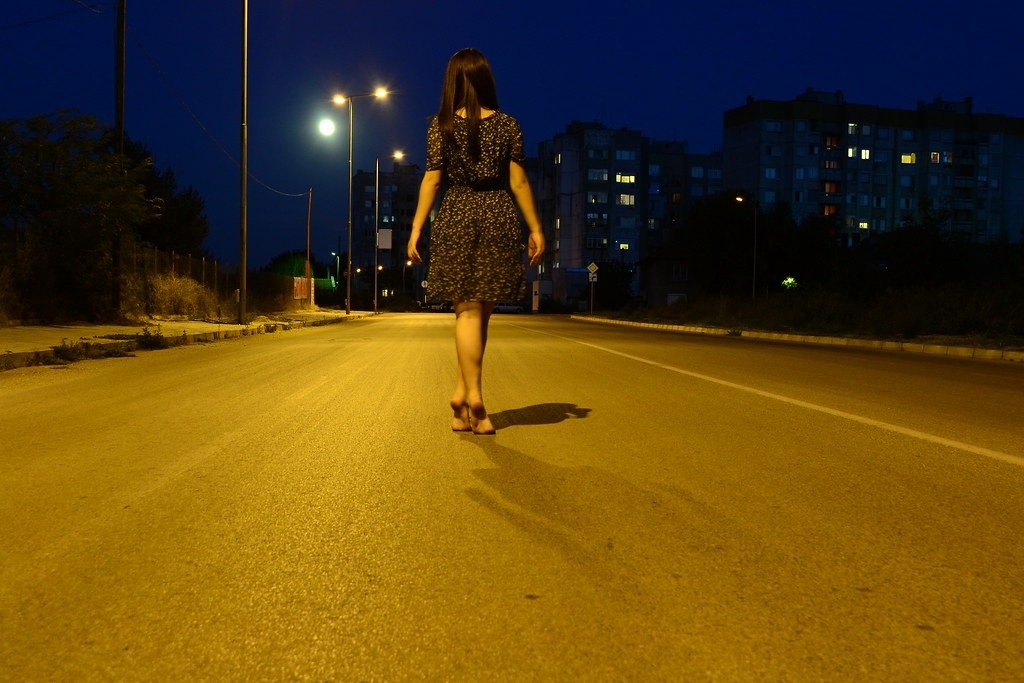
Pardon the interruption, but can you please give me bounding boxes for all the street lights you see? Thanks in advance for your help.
[735,196,757,303]
[374,152,404,313]
[333,88,386,315]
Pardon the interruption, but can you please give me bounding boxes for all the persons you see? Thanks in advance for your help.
[408,47,545,433]
